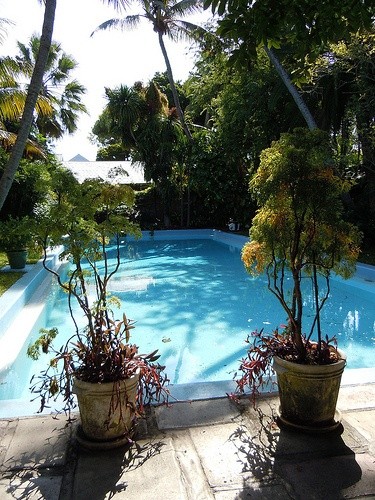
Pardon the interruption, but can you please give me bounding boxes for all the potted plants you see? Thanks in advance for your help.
[0,214,40,269]
[225,127,363,433]
[28,168,178,455]
